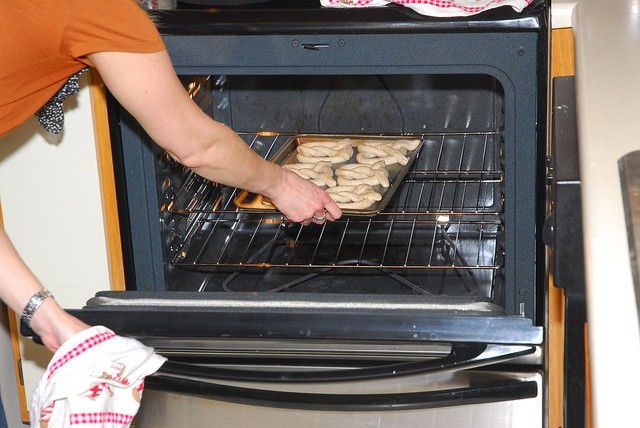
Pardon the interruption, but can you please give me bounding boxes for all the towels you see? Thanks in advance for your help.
[27,326,169,428]
[319,0,535,19]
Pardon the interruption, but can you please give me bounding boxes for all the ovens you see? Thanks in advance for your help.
[103,6,546,385]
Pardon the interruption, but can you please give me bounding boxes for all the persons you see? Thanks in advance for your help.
[1,0,343,427]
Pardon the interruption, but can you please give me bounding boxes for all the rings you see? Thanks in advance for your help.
[312,212,326,221]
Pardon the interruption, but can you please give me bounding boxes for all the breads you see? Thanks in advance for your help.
[356,139,421,166]
[325,183,382,210]
[334,160,390,188]
[281,160,337,188]
[296,137,360,164]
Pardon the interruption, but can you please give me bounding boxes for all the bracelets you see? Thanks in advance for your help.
[20,287,53,327]
